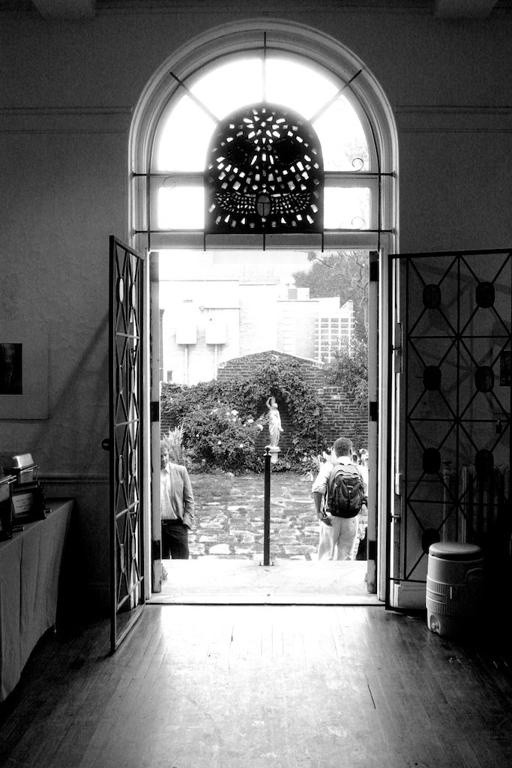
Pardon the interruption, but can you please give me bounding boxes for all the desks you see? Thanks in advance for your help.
[1,497,74,706]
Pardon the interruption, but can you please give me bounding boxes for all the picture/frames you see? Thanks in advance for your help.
[0,317,50,421]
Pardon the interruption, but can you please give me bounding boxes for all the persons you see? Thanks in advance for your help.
[161,442,194,559]
[312,437,370,559]
[265,397,283,448]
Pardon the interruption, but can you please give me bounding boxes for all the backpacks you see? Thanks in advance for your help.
[325,461,363,518]
